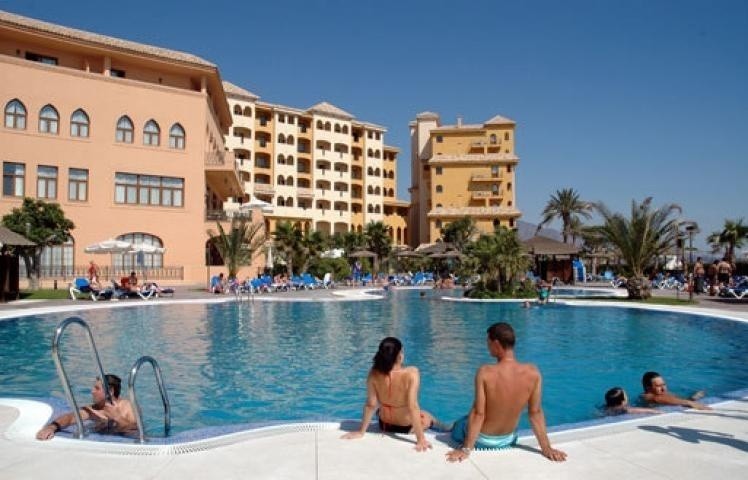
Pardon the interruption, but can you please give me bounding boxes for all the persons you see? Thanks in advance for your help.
[604,372,713,414]
[339,337,442,452]
[446,322,568,464]
[70,260,162,301]
[36,374,138,440]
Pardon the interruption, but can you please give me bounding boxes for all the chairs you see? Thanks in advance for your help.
[207,269,323,294]
[70,279,176,300]
[650,272,746,301]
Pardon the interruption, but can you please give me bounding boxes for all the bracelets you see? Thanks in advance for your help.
[51,421,61,432]
[460,446,471,455]
